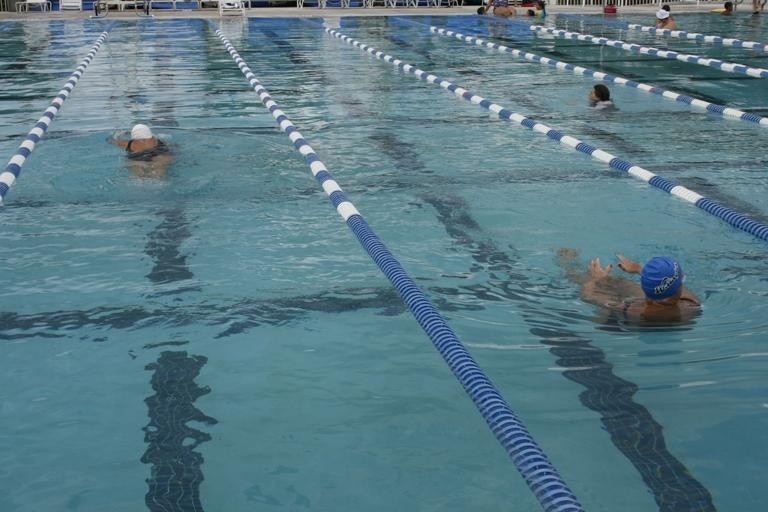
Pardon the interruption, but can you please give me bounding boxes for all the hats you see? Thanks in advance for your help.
[130,123,152,139]
[656,8,670,20]
[641,256,682,300]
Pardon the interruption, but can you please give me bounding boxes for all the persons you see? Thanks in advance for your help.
[476,0,547,19]
[652,9,677,30]
[552,242,707,329]
[655,4,676,22]
[476,11,548,39]
[719,2,735,17]
[105,122,184,192]
[584,84,623,113]
